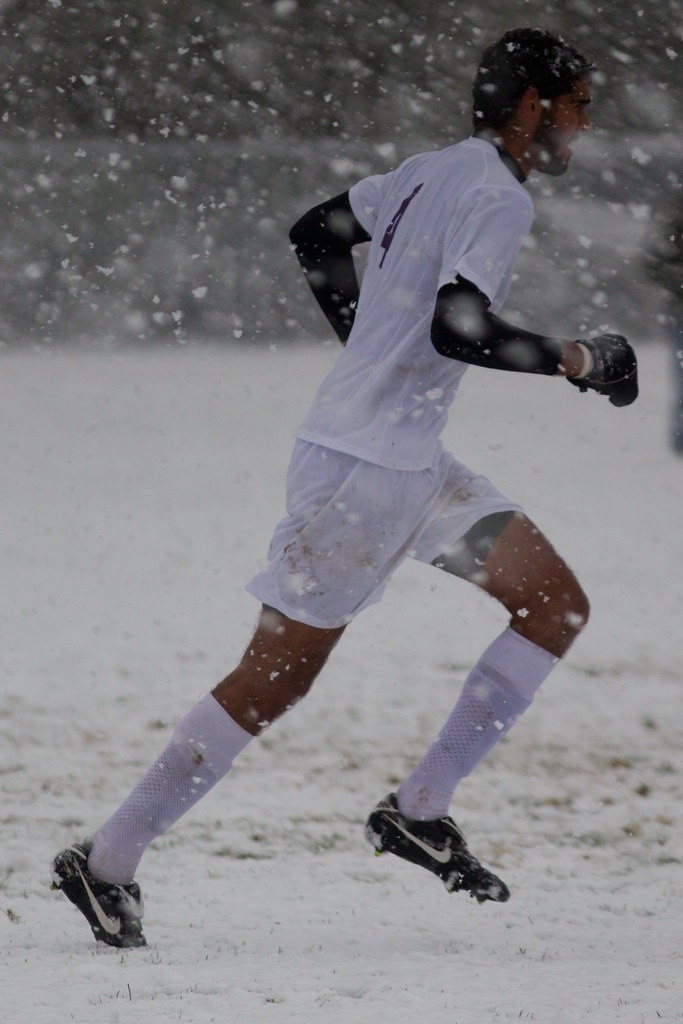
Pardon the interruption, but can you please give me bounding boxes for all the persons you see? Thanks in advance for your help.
[55,30,641,950]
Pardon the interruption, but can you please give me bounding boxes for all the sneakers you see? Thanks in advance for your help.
[49,837,148,949]
[365,792,510,906]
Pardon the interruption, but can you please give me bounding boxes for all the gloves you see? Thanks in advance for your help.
[566,334,638,407]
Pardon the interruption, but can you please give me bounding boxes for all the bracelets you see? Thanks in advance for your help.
[574,344,593,382]
